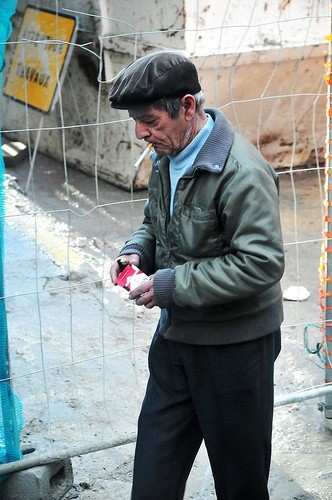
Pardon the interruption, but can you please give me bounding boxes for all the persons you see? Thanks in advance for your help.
[108,50,284,499]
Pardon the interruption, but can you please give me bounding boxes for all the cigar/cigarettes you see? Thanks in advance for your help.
[133,142,153,169]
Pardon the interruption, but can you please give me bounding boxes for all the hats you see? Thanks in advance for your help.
[107,49,202,110]
